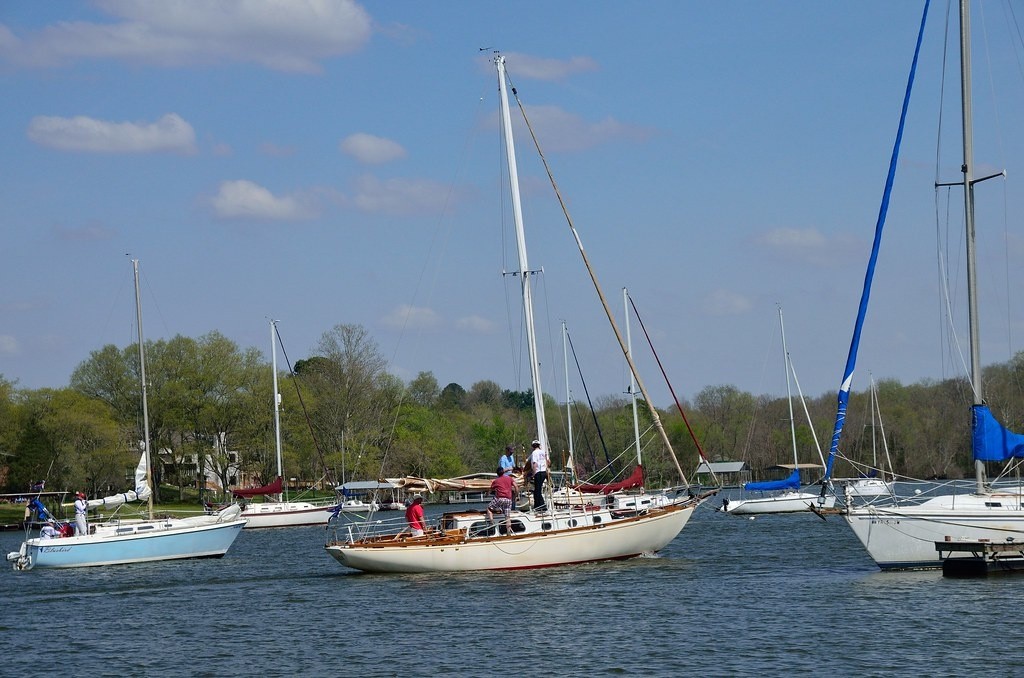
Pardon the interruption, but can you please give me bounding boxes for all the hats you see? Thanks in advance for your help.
[505,446,514,454]
[414,494,423,500]
[76,492,85,498]
[48,518,55,524]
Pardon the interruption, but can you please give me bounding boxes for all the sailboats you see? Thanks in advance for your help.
[543,317,624,506]
[6,253,250,571]
[212,316,348,530]
[717,301,840,515]
[323,45,730,577]
[841,371,898,498]
[802,0,1024,569]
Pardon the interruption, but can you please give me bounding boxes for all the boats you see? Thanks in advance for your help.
[325,430,535,544]
[342,490,380,514]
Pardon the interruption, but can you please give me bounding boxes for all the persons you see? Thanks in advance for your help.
[405,494,427,537]
[531,439,551,511]
[40,518,60,539]
[74,493,88,536]
[486,466,519,535]
[498,446,518,511]
